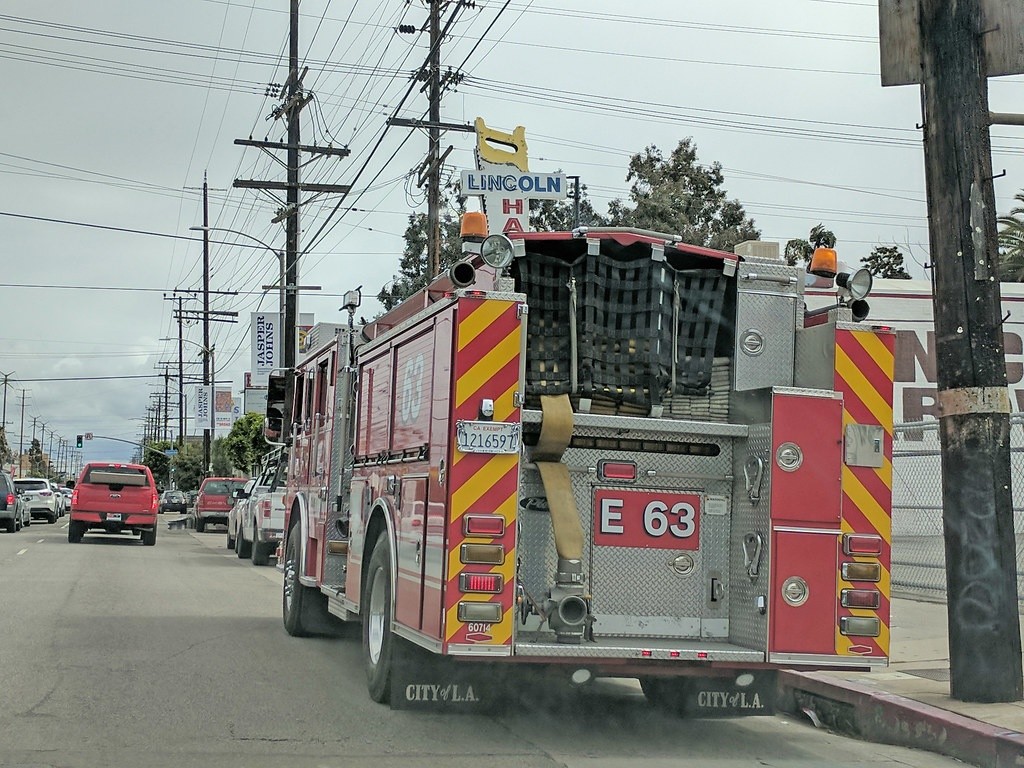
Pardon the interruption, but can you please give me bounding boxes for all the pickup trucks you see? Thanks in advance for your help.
[231,465,327,567]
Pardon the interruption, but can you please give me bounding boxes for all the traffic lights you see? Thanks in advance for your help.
[77,435,82,449]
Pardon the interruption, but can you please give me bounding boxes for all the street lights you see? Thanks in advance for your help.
[159,338,215,454]
[148,383,187,453]
[189,226,285,378]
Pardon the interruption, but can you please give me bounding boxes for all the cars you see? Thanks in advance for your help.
[13,477,73,525]
[192,477,251,533]
[16,487,31,528]
[227,477,257,549]
[158,491,188,516]
[66,462,158,548]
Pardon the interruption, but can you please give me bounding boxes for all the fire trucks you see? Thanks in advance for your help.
[263,209,894,722]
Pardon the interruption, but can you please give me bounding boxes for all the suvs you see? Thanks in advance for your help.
[0,470,22,534]
